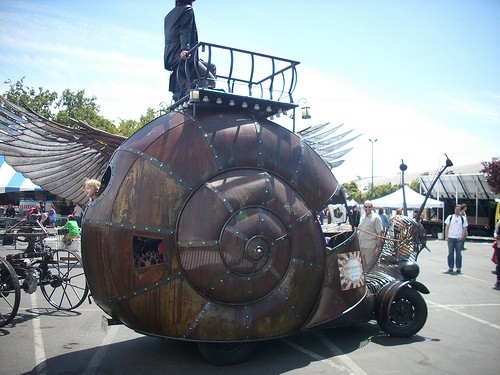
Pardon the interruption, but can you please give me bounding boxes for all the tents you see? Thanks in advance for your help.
[370,184,444,240]
[0,155,44,193]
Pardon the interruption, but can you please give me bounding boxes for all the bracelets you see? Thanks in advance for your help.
[376,244,379,249]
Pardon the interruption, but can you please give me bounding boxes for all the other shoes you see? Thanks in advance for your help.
[444,270,453,274]
[452,271,461,275]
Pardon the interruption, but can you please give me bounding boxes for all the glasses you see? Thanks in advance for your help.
[364,206,372,208]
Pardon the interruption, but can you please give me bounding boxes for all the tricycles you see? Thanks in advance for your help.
[0,218,90,328]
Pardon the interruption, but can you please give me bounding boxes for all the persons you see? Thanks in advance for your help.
[443,204,468,273]
[319,207,423,233]
[358,201,383,270]
[164,0,216,103]
[491,219,500,291]
[2,200,84,247]
[84,179,100,200]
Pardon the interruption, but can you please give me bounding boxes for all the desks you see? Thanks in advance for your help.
[5,233,55,251]
[421,221,446,238]
[0,217,18,228]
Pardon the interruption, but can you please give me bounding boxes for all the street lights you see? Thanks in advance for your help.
[369,138,378,200]
